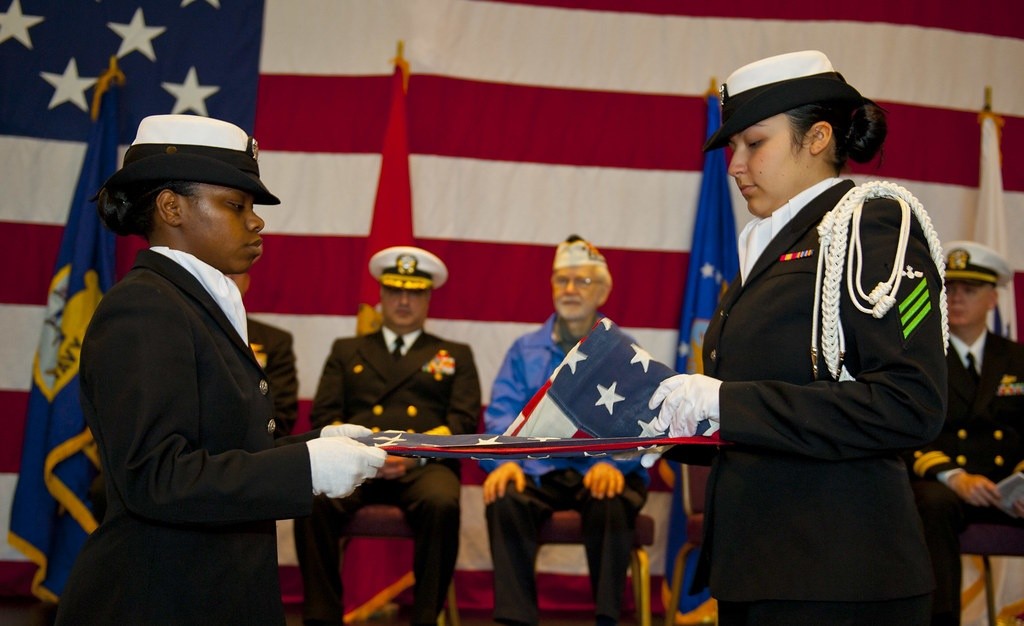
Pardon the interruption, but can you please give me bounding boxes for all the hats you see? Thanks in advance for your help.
[369,246,448,289]
[122,114,280,205]
[702,49,861,152]
[553,235,606,268]
[942,240,1012,286]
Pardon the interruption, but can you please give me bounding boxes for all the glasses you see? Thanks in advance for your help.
[552,278,603,288]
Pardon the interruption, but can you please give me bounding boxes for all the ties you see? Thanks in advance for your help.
[966,352,980,377]
[391,337,404,362]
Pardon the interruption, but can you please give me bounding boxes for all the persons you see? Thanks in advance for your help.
[636,49,950,626]
[294,246,482,626]
[55,114,388,626]
[479,234,651,626]
[898,241,1024,626]
[224,273,298,441]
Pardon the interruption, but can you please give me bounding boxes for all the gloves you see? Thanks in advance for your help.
[305,437,387,498]
[320,424,373,437]
[641,374,723,468]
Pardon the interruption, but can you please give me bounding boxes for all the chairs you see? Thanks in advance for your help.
[334,504,460,626]
[666,464,709,626]
[536,510,655,626]
[960,525,1024,626]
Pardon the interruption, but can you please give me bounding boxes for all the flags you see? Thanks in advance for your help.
[973,86,1017,342]
[357,41,415,338]
[7,56,125,602]
[661,77,743,622]
